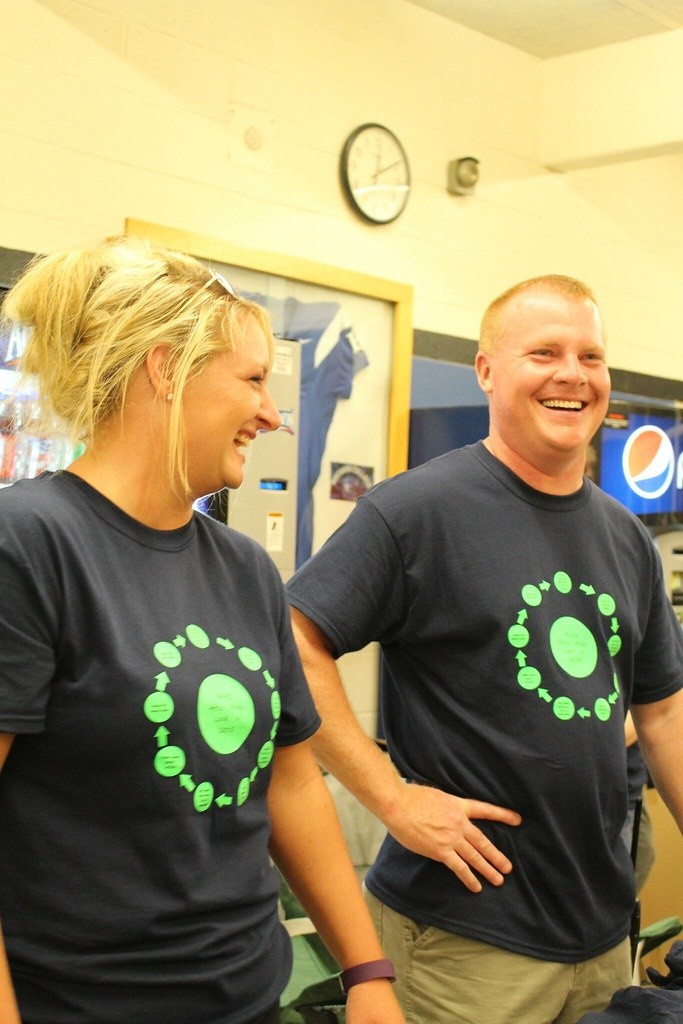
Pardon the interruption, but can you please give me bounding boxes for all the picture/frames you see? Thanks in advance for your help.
[123,215,417,594]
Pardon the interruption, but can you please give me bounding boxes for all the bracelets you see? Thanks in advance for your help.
[338,958,397,993]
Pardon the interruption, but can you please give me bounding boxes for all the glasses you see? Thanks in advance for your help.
[173,269,245,315]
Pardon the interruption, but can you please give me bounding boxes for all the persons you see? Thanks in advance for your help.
[288,273,683,1024]
[1,235,409,1024]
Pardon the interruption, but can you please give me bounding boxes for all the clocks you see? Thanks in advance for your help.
[340,123,412,224]
